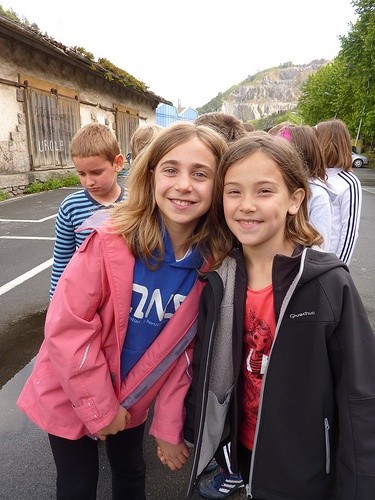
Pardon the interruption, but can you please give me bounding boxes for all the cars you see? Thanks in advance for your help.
[351,150,370,167]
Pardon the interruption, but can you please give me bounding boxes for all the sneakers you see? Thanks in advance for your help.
[198,471,244,500]
[205,457,219,471]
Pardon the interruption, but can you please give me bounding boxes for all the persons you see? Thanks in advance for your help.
[16,123,225,500]
[49,123,129,302]
[130,113,362,500]
[157,136,375,500]
